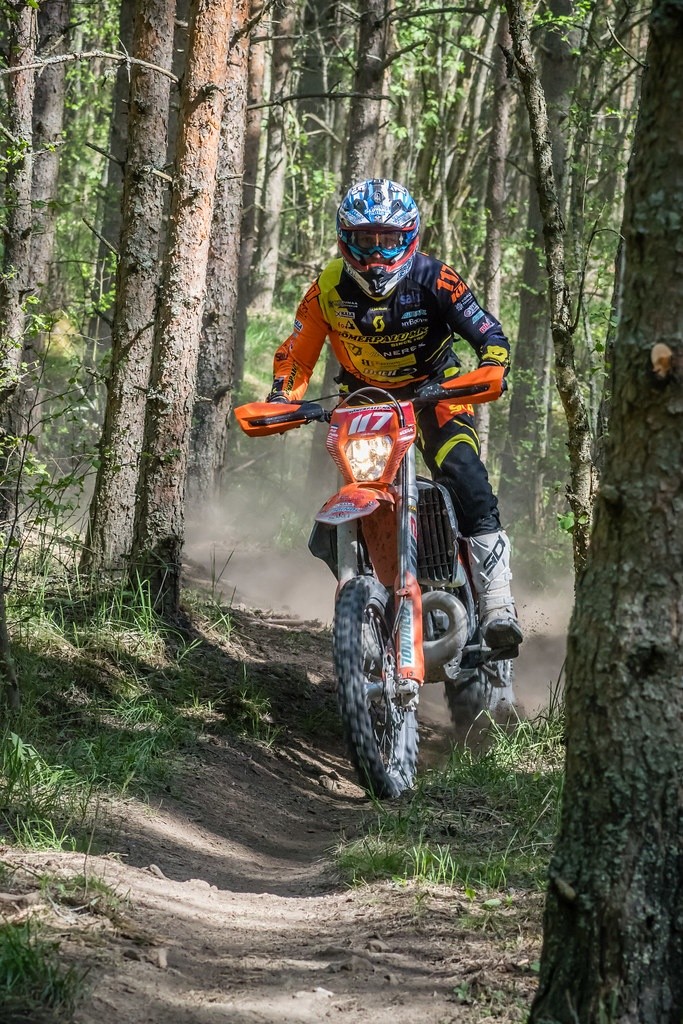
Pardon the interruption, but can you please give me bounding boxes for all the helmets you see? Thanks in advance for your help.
[337,175,422,300]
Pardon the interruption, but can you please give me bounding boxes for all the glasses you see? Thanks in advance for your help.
[335,224,417,262]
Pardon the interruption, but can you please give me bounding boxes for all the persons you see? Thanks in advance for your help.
[254,180,522,661]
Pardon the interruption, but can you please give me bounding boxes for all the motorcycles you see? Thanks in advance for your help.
[234,367,514,803]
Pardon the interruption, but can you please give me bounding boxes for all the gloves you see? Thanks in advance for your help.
[481,361,507,397]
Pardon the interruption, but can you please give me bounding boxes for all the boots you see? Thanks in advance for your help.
[466,530,522,659]
